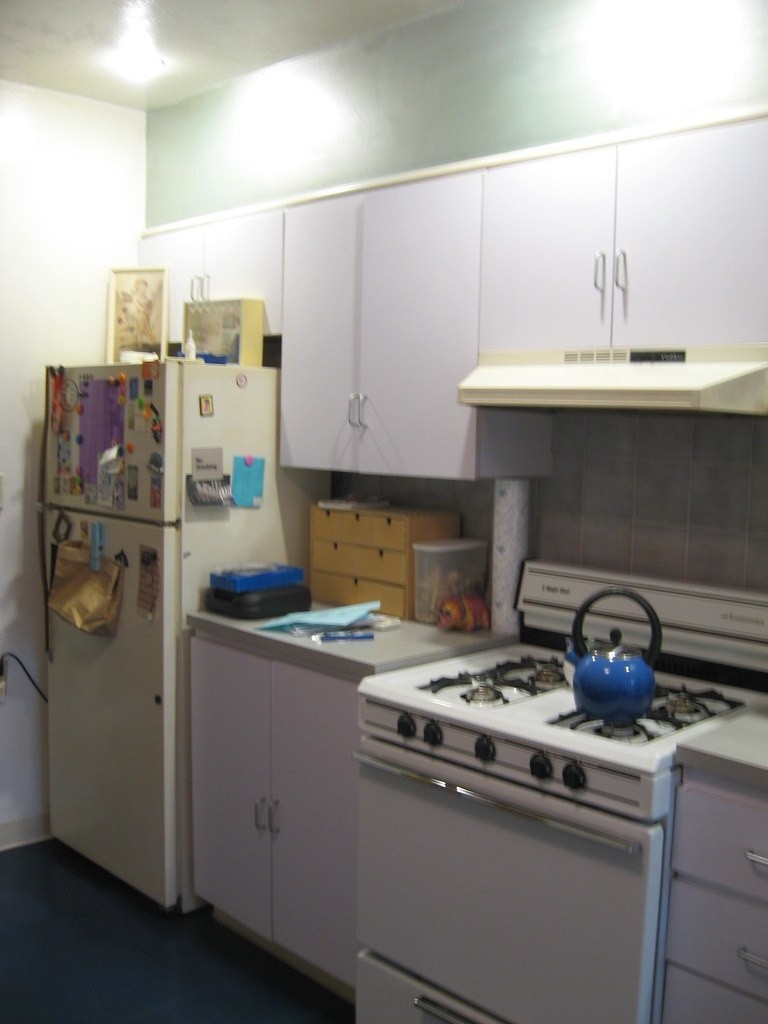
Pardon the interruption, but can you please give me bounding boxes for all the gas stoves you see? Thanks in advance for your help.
[357,561,768,824]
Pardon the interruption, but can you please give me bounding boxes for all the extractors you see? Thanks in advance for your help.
[457,343,768,415]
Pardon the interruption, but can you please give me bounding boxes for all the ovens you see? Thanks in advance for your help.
[353,735,681,1024]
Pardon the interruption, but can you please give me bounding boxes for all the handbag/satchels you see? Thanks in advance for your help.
[49,541,125,637]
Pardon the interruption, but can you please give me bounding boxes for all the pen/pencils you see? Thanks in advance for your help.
[320,633,374,640]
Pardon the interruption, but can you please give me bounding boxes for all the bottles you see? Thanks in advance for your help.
[185,330,195,363]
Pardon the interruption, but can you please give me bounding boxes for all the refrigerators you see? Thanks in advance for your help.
[43,362,329,913]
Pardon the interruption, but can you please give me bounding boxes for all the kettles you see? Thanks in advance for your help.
[567,589,662,727]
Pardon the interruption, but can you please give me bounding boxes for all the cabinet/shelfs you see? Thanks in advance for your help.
[186,601,491,1007]
[310,502,461,621]
[279,169,482,481]
[140,208,285,341]
[479,115,768,351]
[663,702,768,1024]
